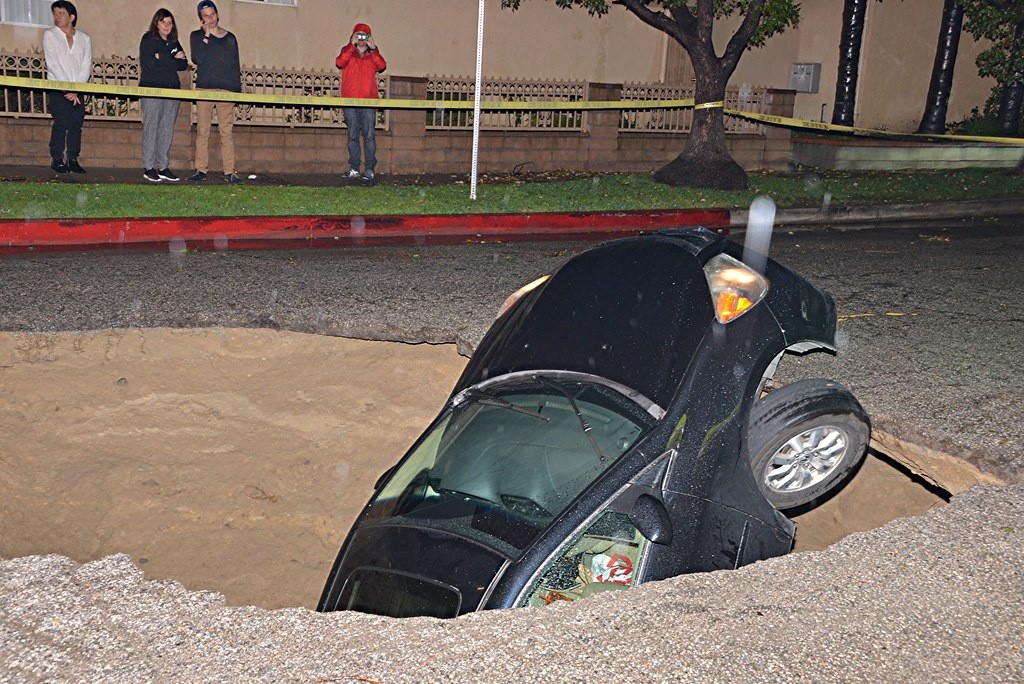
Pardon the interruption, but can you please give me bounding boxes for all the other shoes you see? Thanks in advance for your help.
[51,158,70,173]
[66,157,87,173]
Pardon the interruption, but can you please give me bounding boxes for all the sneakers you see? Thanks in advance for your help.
[142,168,162,182]
[362,169,374,180]
[185,171,207,182]
[340,168,361,178]
[157,168,180,182]
[224,173,244,185]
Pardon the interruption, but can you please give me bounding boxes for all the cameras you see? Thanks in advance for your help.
[355,33,369,41]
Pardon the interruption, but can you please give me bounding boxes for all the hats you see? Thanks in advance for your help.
[353,23,372,37]
[198,1,216,17]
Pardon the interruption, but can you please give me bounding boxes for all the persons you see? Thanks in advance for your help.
[43,0,92,174]
[336,23,387,180]
[185,0,241,182]
[138,8,188,182]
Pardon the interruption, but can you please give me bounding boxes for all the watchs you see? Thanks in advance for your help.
[203,35,209,39]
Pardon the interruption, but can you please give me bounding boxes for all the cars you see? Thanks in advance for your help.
[315,223,872,620]
